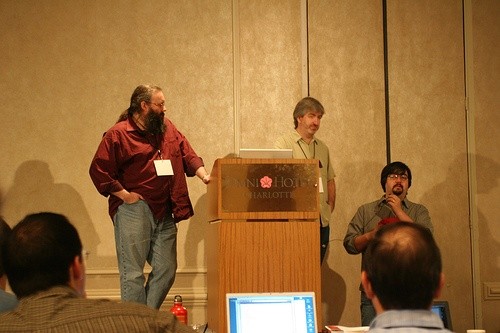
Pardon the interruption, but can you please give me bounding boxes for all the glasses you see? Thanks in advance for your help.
[147,101,164,109]
[388,173,408,181]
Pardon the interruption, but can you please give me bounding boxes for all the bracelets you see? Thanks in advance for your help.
[201,173,209,182]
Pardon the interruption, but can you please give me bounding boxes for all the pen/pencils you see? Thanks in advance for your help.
[203,323,208,333]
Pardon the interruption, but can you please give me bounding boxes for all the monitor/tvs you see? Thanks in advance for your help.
[224,291,317,333]
[431,300,453,330]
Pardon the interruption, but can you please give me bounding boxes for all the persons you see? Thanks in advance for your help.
[0,212,177,333]
[273,96,336,266]
[343,158,433,326]
[89,83,211,311]
[361,221,451,333]
